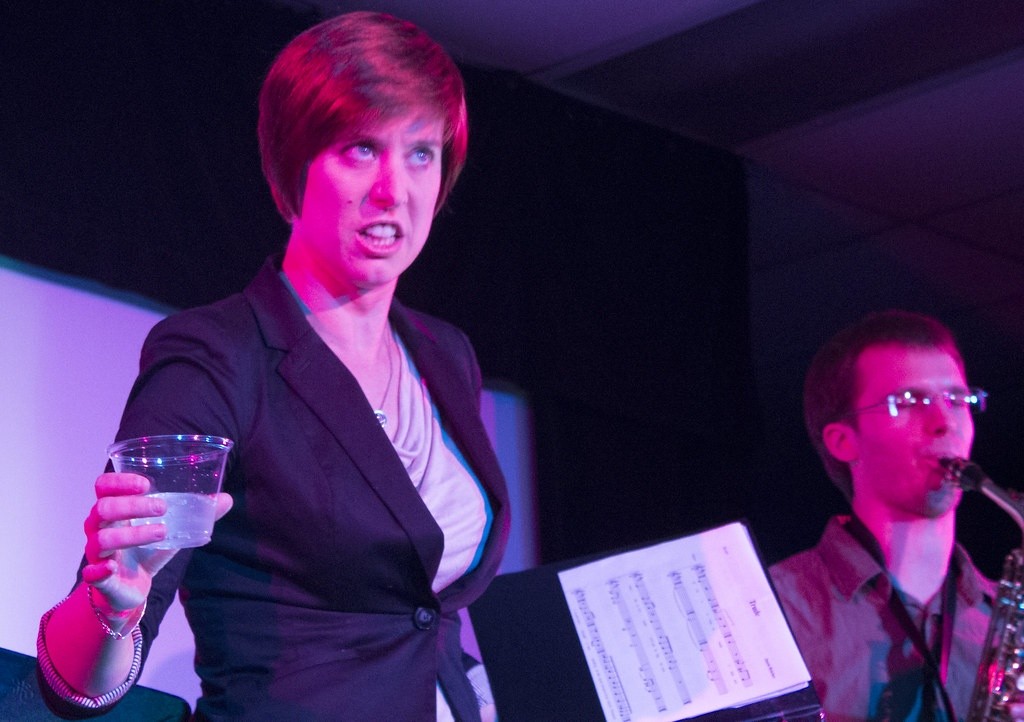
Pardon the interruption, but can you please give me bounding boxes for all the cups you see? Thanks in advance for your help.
[107,434,235,549]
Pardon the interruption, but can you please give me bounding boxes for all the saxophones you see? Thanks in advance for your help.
[939,457,1024,722]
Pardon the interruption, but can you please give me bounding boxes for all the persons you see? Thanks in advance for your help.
[766,311,1024,722]
[35,11,513,722]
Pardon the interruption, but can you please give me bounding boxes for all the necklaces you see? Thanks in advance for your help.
[371,320,394,426]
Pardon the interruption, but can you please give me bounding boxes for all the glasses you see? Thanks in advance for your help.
[855,388,987,418]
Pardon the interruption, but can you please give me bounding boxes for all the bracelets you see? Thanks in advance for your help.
[87,583,147,641]
